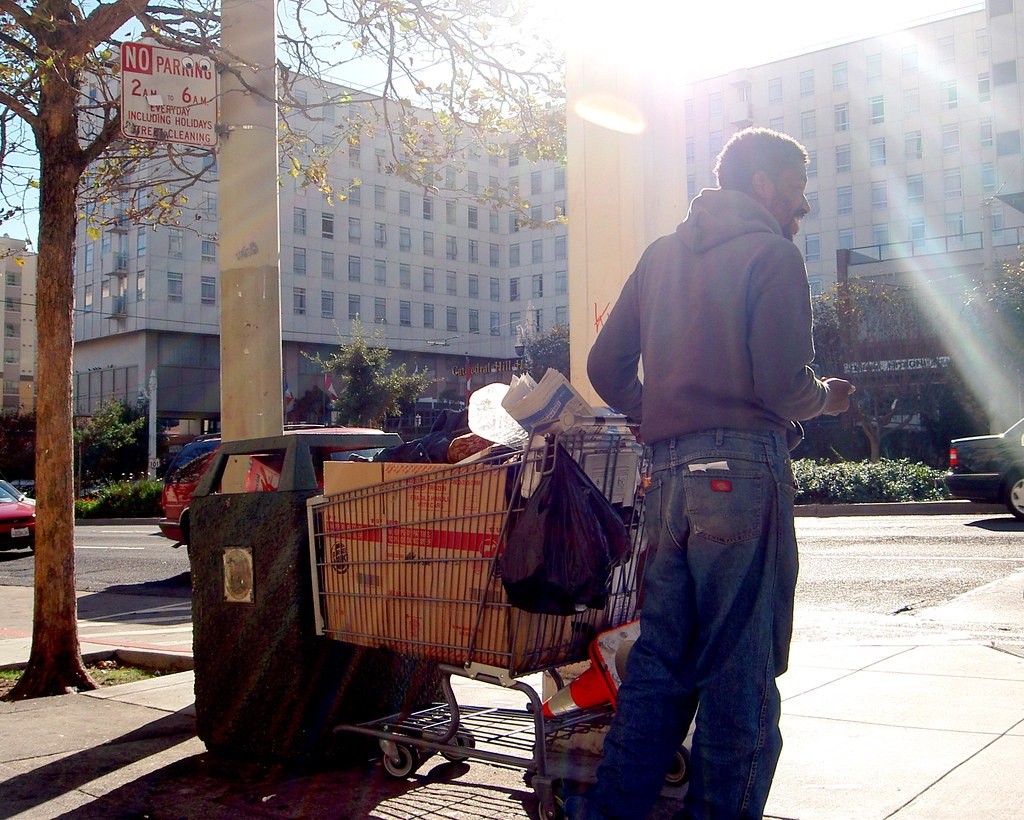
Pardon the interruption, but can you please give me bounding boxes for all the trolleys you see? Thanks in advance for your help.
[306,413,691,820]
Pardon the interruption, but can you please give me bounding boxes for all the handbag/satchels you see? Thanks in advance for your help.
[498,436,631,617]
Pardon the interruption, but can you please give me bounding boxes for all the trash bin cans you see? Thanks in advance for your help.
[181,425,450,779]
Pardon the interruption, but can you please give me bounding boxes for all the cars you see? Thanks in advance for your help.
[157,422,354,549]
[943,416,1024,521]
[0,480,36,550]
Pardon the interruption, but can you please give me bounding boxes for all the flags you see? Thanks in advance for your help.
[324,373,339,400]
[465,359,471,398]
[284,379,294,404]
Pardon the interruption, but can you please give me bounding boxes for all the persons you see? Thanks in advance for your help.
[585,126,857,820]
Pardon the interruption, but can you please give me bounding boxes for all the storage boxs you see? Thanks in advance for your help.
[320,459,573,670]
